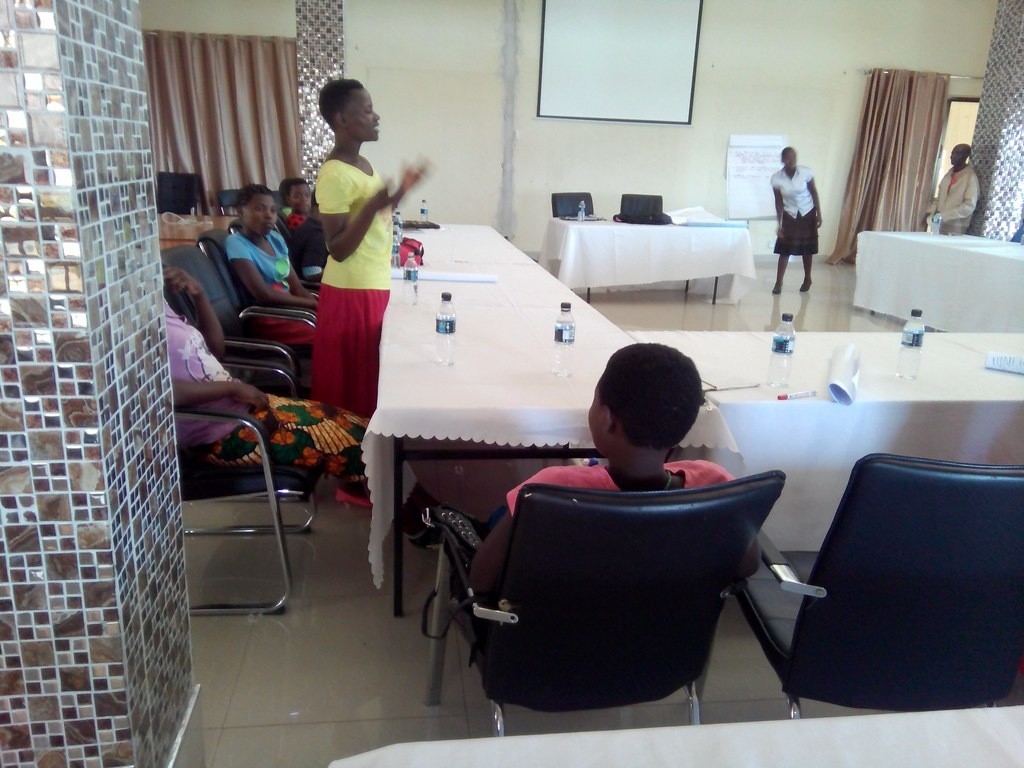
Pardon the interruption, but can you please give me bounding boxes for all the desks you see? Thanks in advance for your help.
[330,706,1023,768]
[360,225,745,614]
[854,231,1024,335]
[629,332,1024,546]
[538,217,756,307]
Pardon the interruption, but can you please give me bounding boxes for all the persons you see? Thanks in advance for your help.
[279,179,328,282]
[924,143,980,234]
[312,77,424,507]
[467,343,761,594]
[224,184,318,344]
[771,146,822,294]
[164,266,490,551]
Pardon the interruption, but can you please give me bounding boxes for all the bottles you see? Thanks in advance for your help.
[930,211,941,236]
[553,302,576,378]
[401,252,419,306]
[577,200,585,222]
[894,309,925,379]
[766,313,796,389]
[435,291,457,364]
[390,205,403,279]
[419,199,431,222]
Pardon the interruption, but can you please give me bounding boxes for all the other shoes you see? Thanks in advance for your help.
[335,487,372,507]
[800,280,812,291]
[407,525,445,551]
[772,282,782,294]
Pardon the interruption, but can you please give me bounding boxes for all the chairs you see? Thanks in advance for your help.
[420,466,790,739]
[621,194,662,217]
[553,192,595,221]
[157,173,325,620]
[738,455,1024,720]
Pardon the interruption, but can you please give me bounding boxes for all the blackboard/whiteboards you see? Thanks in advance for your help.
[535,0,703,126]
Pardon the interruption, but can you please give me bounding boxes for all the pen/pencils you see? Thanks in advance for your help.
[777,390,816,400]
